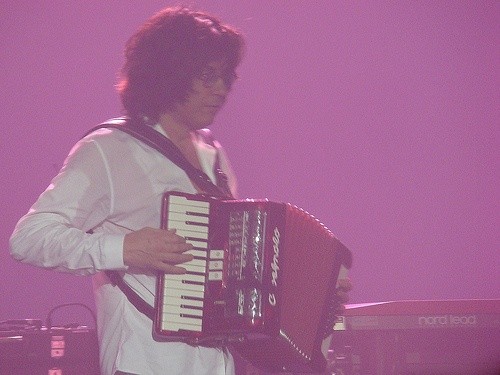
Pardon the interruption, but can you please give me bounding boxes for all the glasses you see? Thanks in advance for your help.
[197,70,240,88]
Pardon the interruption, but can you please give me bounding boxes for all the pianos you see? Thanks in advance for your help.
[334,297,500,375]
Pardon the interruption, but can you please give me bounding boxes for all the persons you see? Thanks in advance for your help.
[10,8,351,375]
